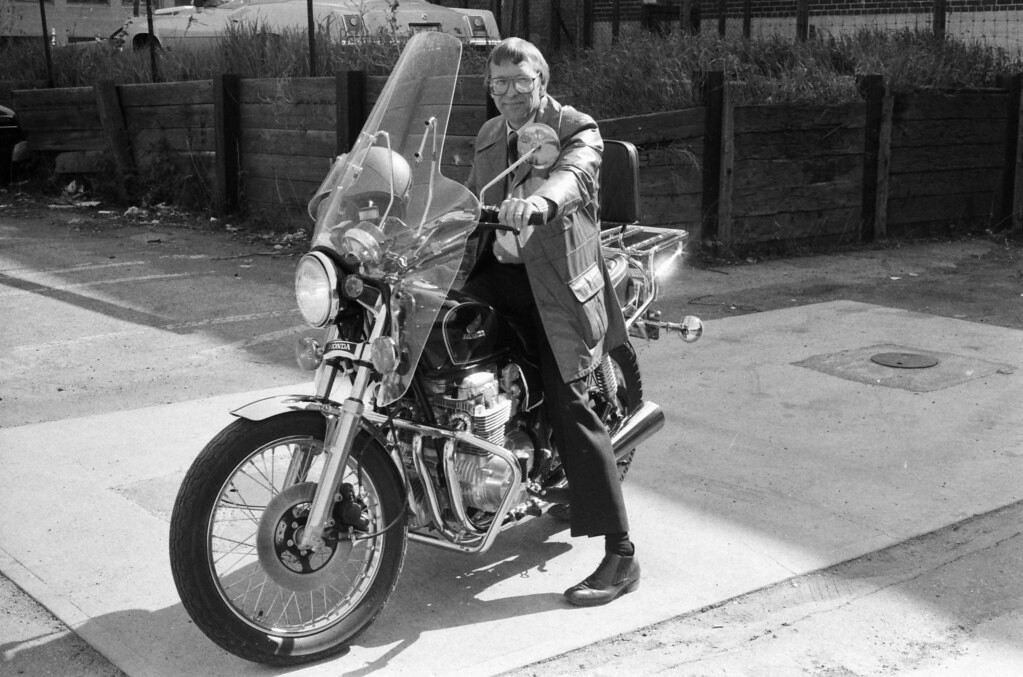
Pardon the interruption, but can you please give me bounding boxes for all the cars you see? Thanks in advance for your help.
[120,0,473,56]
[449,7,503,51]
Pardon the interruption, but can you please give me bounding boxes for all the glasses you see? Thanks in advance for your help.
[488,72,542,96]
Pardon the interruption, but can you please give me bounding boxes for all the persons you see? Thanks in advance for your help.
[462,37,641,606]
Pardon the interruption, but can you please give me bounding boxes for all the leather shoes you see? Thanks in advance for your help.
[563,542,641,606]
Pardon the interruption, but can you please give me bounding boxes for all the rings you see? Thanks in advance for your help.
[515,212,522,215]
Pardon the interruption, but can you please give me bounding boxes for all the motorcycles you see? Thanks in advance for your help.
[170,32,704,668]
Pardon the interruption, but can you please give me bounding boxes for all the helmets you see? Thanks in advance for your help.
[309,146,412,234]
